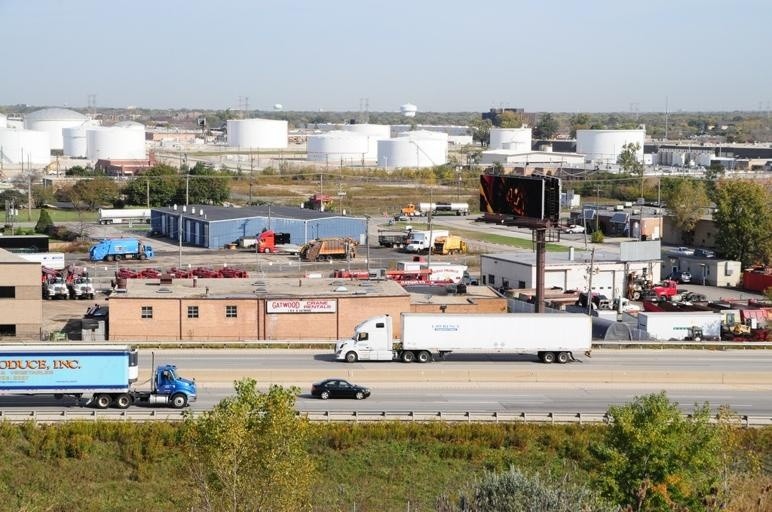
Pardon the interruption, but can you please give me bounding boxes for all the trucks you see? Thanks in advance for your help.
[301,236,359,263]
[400,201,471,216]
[40,265,70,301]
[89,237,153,263]
[1,342,198,409]
[508,286,610,312]
[651,278,708,303]
[98,201,242,226]
[333,310,594,363]
[404,228,449,254]
[432,235,467,256]
[256,230,303,255]
[65,266,97,300]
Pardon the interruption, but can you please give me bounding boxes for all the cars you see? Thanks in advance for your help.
[666,244,718,258]
[563,223,588,235]
[310,377,372,401]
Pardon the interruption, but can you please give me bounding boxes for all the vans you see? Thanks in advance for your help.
[666,271,692,284]
